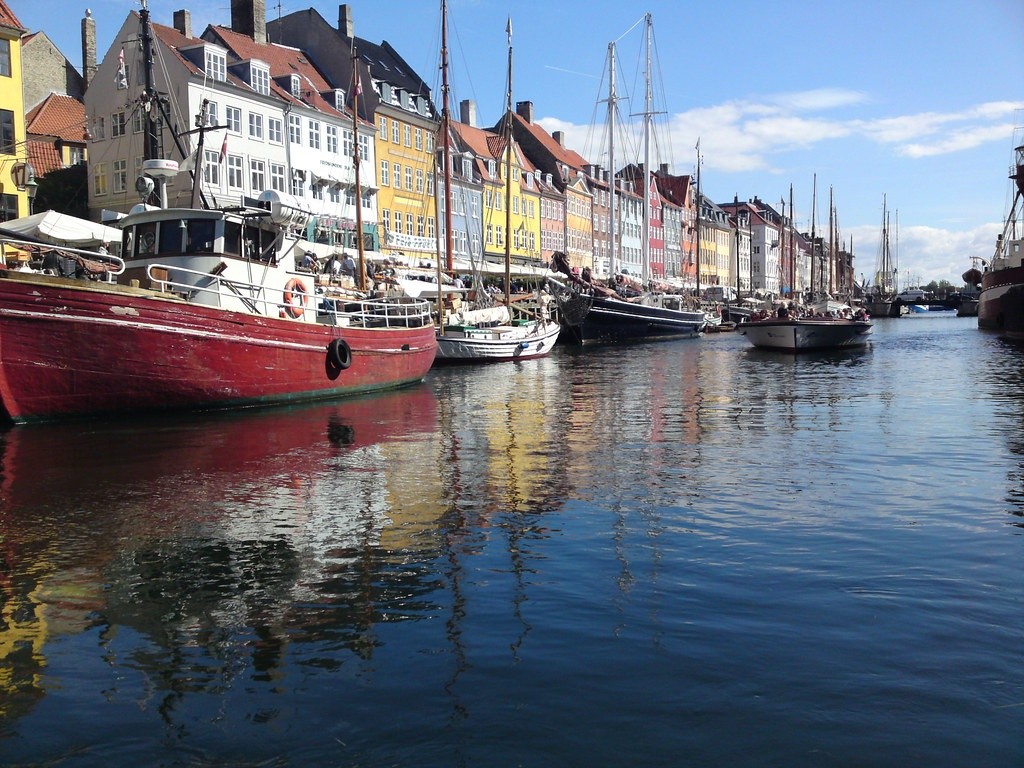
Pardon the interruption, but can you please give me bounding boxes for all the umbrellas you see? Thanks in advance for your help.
[0,210,134,247]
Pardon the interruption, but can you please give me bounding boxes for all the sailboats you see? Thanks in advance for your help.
[340,0,1024,364]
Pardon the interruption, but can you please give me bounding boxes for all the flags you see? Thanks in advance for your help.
[114,49,128,90]
[178,145,206,172]
[219,133,228,163]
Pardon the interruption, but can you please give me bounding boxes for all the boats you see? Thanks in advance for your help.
[1,2,438,427]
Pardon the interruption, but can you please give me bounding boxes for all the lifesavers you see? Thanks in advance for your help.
[716,306,721,316]
[331,339,354,368]
[282,278,310,320]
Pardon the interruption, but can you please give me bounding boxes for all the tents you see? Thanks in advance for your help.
[623,273,712,290]
[293,240,568,279]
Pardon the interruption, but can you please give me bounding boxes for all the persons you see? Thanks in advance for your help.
[302,250,432,288]
[463,275,641,297]
[753,287,868,321]
[28,248,63,276]
